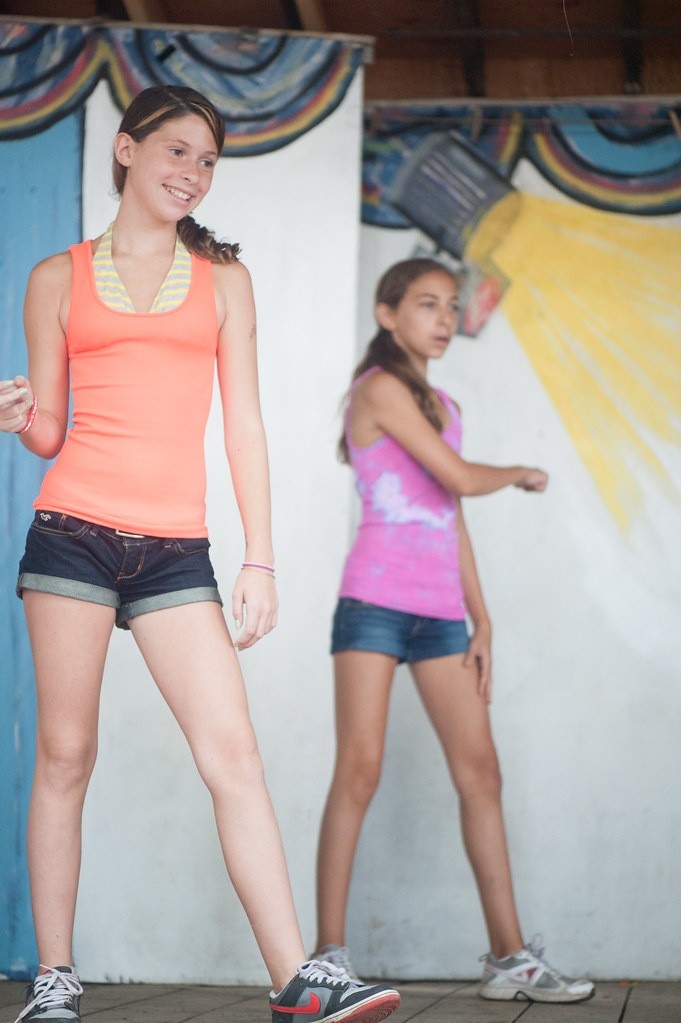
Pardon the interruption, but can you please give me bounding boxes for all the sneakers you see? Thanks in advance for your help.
[269,959,402,1022]
[309,943,358,981]
[478,934,597,1005]
[13,963,83,1023]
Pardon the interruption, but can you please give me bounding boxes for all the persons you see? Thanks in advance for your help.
[306,257,599,1006]
[1,84,403,1023]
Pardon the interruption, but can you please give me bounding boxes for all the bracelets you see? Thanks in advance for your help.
[240,561,275,578]
[16,392,38,434]
